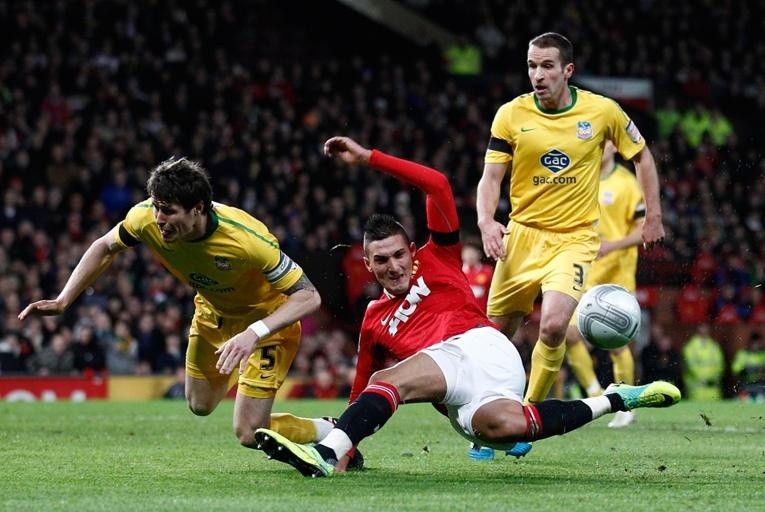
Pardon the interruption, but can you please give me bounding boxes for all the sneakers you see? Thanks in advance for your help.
[467,448,495,460]
[254,416,364,477]
[603,381,682,428]
[505,441,532,457]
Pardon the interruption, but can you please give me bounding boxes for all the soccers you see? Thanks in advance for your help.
[576,282,640,349]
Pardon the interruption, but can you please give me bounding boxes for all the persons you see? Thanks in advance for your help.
[468,32,666,460]
[565,137,646,427]
[17,156,364,471]
[0,0,765,401]
[253,135,682,477]
